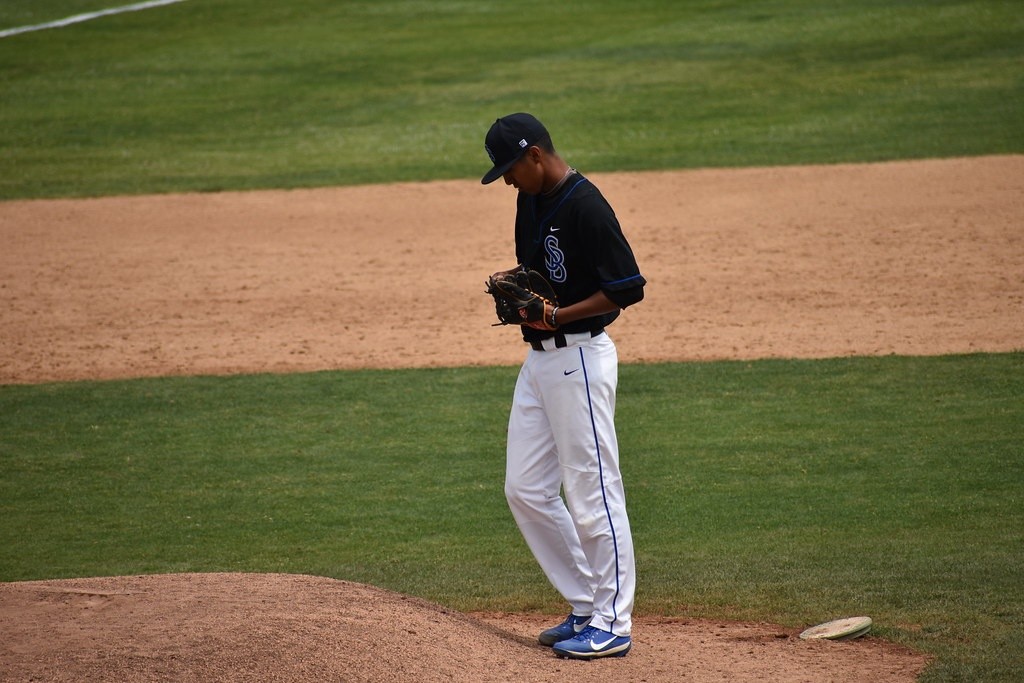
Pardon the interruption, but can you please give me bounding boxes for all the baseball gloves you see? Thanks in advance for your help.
[483,263,563,334]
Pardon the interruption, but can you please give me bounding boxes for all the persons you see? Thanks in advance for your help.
[480,112,647,659]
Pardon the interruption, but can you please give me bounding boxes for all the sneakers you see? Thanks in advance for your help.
[553,625,631,658]
[539,611,594,645]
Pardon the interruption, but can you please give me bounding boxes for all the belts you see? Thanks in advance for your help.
[531,328,603,351]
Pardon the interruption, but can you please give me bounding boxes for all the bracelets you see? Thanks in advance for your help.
[551,306,559,325]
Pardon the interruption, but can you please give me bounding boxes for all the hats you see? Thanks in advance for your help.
[481,113,547,184]
